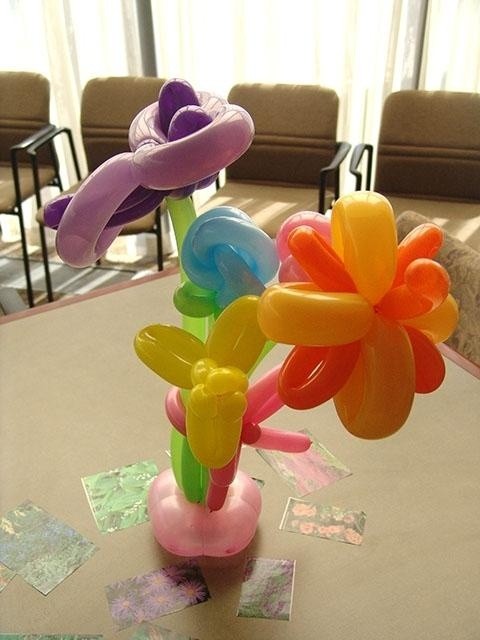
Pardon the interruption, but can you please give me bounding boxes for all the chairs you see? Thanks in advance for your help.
[26,75,180,307]
[196,83,352,242]
[396,205,480,365]
[348,92,479,254]
[0,71,64,314]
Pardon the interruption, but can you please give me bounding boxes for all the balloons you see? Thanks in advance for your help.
[44,75,459,561]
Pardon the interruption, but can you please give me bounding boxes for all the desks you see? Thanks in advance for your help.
[0,255,479,637]
